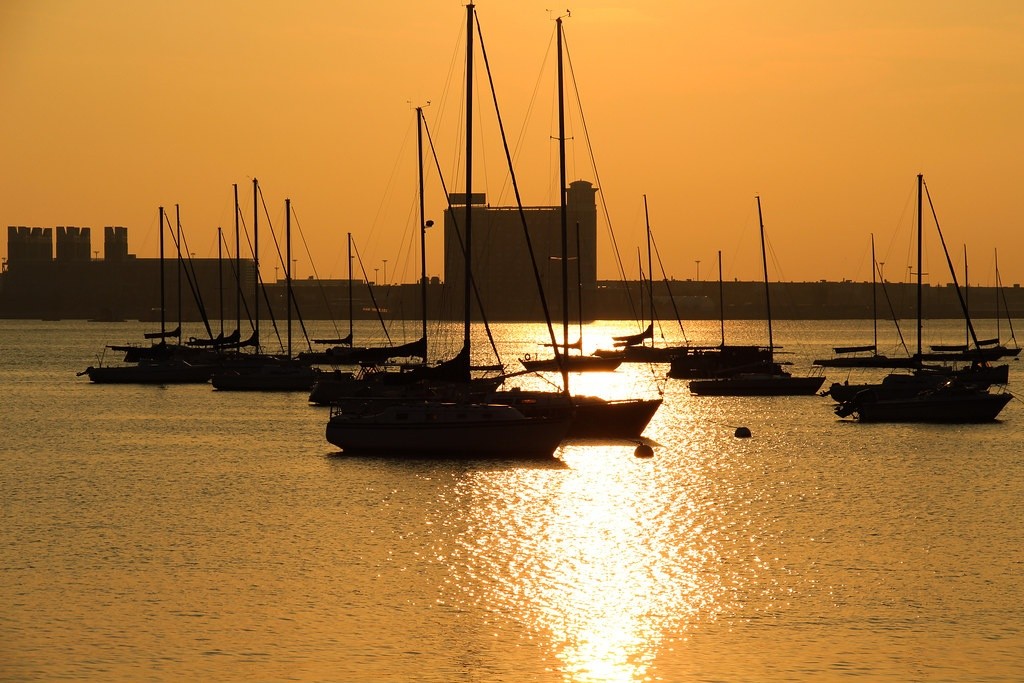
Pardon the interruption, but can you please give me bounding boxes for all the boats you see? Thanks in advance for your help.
[828,174,1011,423]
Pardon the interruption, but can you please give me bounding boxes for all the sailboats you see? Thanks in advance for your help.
[666,250,791,380]
[85,3,662,438]
[690,197,828,397]
[811,171,1022,366]
[326,107,576,461]
[596,193,785,378]
